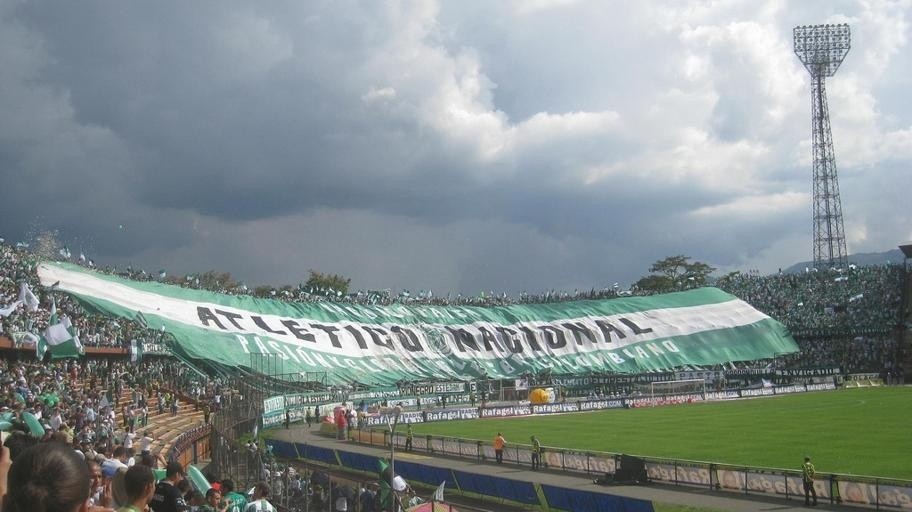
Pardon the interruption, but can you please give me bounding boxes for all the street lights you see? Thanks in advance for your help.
[380,404,403,511]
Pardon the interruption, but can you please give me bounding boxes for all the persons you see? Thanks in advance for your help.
[494,431,508,464]
[529,434,539,471]
[800,457,818,505]
[0,239,423,511]
[424,261,910,408]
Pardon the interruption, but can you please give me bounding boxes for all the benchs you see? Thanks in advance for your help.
[59,376,208,459]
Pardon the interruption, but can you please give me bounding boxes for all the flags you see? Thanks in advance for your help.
[431,479,447,503]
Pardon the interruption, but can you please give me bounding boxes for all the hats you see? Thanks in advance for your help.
[167,461,188,476]
